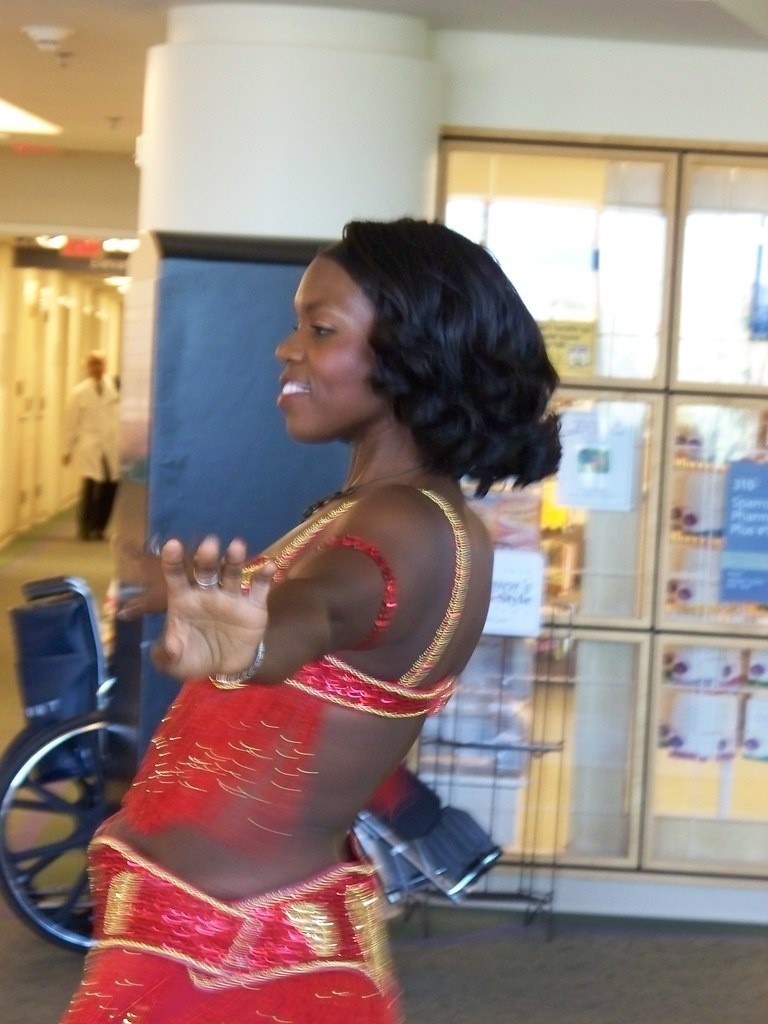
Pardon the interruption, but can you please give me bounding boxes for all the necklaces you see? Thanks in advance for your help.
[297,464,427,521]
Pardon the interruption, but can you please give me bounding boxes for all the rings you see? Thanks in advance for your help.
[193,568,220,590]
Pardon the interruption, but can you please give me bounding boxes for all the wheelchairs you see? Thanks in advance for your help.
[0,576,500,957]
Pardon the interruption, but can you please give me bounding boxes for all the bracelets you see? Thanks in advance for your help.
[208,640,265,685]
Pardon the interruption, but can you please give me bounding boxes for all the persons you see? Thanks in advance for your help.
[55,217,564,1024]
[61,351,123,540]
